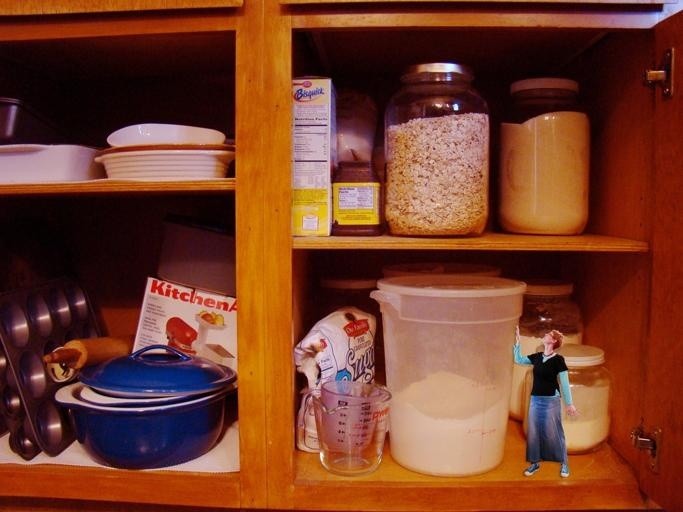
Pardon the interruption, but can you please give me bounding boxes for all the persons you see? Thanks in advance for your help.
[515,324,579,478]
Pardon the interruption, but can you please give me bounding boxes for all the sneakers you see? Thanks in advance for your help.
[560,463,569,477]
[524,463,538,476]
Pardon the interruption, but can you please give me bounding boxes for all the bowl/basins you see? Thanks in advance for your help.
[0,94,234,182]
[53,346,236,471]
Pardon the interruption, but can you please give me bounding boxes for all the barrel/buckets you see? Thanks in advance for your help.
[368,273,528,480]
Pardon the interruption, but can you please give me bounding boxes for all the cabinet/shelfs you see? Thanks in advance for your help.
[0,2,683,511]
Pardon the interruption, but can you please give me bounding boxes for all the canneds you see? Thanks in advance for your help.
[331,160,385,236]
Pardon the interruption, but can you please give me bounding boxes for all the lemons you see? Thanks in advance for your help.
[199,310,225,326]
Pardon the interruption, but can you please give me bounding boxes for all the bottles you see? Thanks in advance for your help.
[385,63,487,235]
[496,78,593,237]
[314,267,452,434]
[496,267,583,426]
[526,338,608,453]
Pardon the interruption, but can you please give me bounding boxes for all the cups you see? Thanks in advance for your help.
[312,388,391,476]
